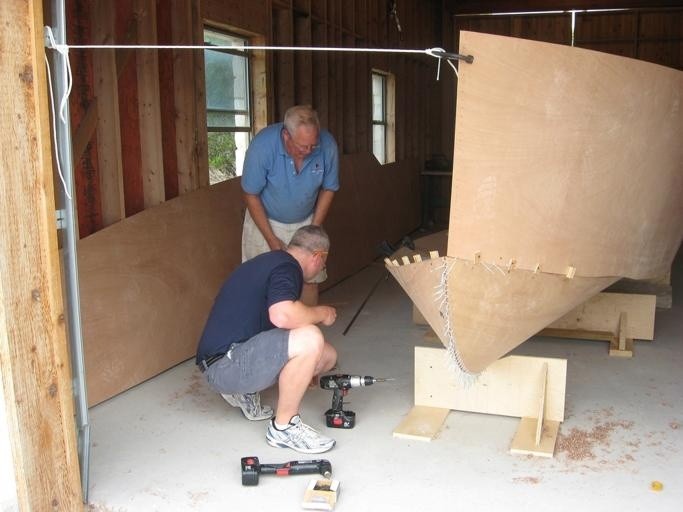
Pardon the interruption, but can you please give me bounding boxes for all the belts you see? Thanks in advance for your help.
[199,354,226,374]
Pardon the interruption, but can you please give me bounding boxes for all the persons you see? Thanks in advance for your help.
[194,225,338,454]
[240,104,342,391]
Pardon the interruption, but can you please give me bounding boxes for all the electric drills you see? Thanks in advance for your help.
[320,374,395,428]
[241,456,332,486]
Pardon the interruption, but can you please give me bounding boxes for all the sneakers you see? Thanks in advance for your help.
[217,392,273,420]
[265,415,336,454]
